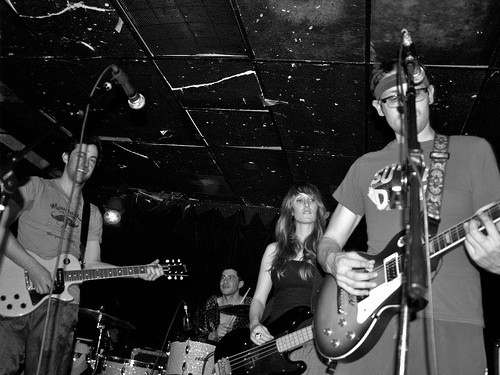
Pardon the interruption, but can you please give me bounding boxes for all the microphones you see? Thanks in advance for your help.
[183,304,190,327]
[111,64,146,109]
[403,28,425,83]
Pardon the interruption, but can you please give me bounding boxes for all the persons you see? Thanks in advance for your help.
[197,263,253,342]
[317,54,500,375]
[248,183,347,347]
[0,133,164,375]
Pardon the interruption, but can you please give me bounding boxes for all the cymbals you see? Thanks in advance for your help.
[216,305,251,316]
[77,307,135,329]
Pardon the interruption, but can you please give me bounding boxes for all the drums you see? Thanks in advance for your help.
[163,334,218,375]
[97,354,163,375]
[123,345,168,365]
[70,338,95,375]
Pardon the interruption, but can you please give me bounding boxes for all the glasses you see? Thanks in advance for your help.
[381,88,428,108]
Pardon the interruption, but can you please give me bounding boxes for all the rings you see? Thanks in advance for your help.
[255,332,262,340]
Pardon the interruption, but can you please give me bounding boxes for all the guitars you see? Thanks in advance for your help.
[213,305,315,375]
[313,199,500,363]
[0,248,190,317]
